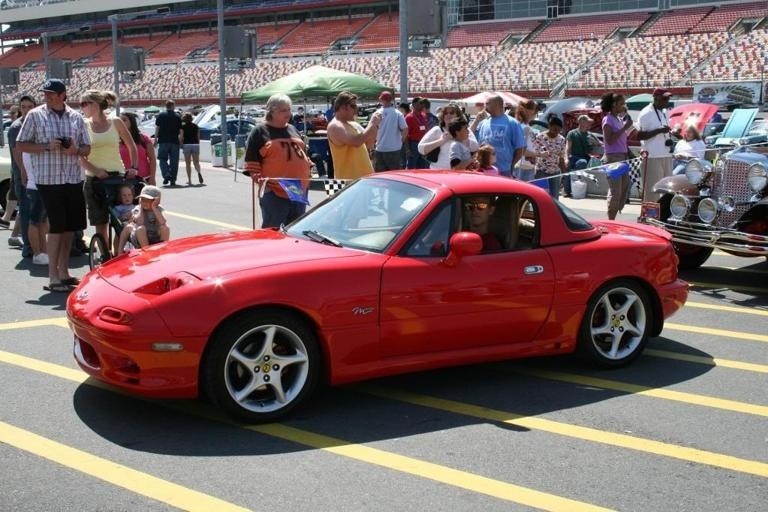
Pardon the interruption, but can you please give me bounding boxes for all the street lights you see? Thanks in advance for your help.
[0,39,37,130]
[108,5,173,117]
[38,26,93,99]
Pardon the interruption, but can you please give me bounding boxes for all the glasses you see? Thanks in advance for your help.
[443,111,457,115]
[463,202,489,212]
[78,100,95,108]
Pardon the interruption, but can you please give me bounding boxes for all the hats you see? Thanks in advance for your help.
[577,114,595,124]
[38,77,67,95]
[134,184,162,200]
[379,91,393,101]
[653,86,673,98]
[20,94,36,103]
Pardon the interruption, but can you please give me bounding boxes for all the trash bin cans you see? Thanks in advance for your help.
[235,134,249,170]
[308,137,327,161]
[211,133,232,168]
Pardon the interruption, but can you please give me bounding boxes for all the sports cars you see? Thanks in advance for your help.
[62,169,695,425]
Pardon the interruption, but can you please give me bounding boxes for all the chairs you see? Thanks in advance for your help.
[488,196,522,250]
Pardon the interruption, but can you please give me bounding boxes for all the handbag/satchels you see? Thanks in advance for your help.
[421,125,444,164]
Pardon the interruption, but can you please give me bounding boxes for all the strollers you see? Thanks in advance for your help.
[88,170,169,273]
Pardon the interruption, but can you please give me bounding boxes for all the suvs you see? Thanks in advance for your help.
[647,133,768,282]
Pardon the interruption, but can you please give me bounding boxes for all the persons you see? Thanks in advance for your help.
[326,92,594,225]
[0,78,171,293]
[241,93,313,227]
[434,195,505,254]
[599,89,707,220]
[178,112,204,186]
[293,106,303,121]
[153,100,181,186]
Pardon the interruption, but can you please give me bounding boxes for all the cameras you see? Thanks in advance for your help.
[56,137,71,149]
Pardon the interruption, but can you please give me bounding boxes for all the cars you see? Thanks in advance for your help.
[0,131,20,218]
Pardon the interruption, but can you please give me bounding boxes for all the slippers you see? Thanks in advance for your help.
[60,276,82,286]
[42,282,77,293]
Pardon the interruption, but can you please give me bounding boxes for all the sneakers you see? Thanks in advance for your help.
[198,172,204,184]
[7,236,25,247]
[170,180,176,187]
[69,245,83,257]
[76,240,91,253]
[0,218,11,229]
[163,179,169,184]
[32,250,52,265]
